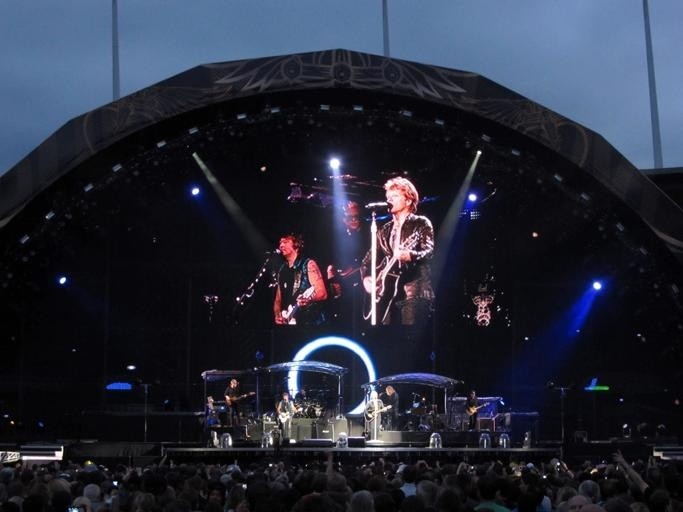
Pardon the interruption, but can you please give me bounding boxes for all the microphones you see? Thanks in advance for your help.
[265,249,281,255]
[365,202,391,210]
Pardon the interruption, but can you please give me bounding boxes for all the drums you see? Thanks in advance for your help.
[304,404,317,419]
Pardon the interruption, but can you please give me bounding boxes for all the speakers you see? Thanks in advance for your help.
[348,438,367,448]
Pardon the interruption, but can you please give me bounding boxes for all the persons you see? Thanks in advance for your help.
[359,174,436,326]
[326,199,370,281]
[204,378,488,432]
[0,452,680,511]
[272,229,329,327]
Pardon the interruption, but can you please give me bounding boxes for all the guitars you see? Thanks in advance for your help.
[278,407,303,424]
[226,392,256,407]
[361,224,432,322]
[329,258,360,300]
[466,403,490,416]
[364,405,392,423]
[273,285,318,343]
